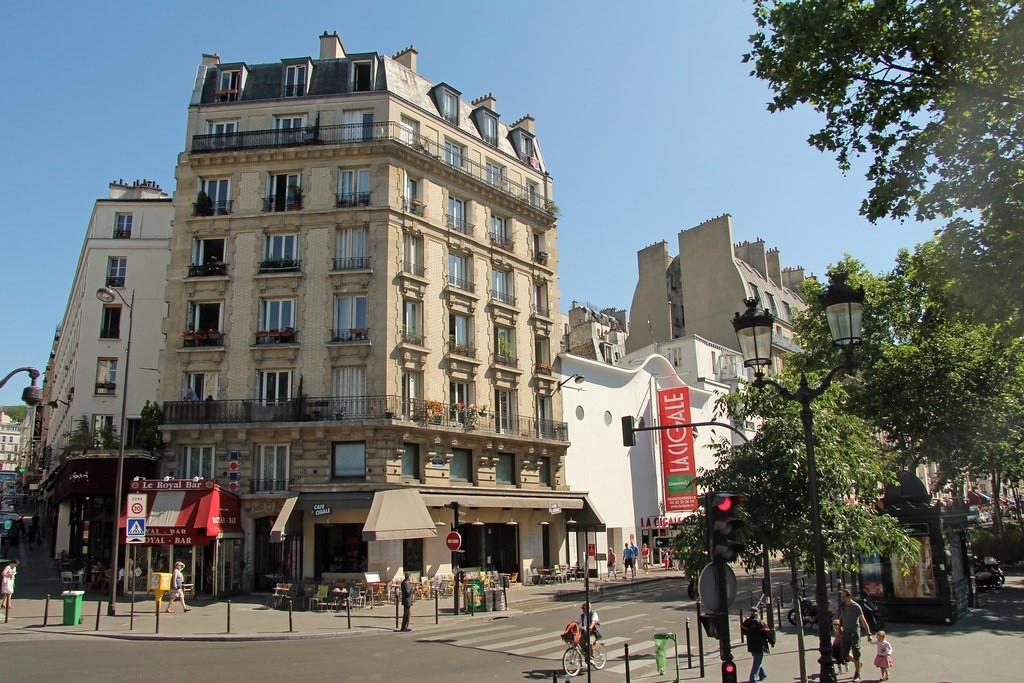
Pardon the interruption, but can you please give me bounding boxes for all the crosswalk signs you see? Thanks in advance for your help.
[127,518,146,537]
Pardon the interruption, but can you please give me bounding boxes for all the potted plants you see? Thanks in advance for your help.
[256,327,295,337]
[183,328,221,340]
[288,184,303,211]
[192,191,228,215]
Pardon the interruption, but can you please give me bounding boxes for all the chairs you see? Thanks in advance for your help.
[61,572,78,591]
[269,564,584,612]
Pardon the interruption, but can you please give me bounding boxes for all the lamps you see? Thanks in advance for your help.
[558,373,585,393]
[435,506,446,526]
[472,507,485,526]
[506,508,518,525]
[47,399,69,409]
[537,508,550,525]
[566,517,577,524]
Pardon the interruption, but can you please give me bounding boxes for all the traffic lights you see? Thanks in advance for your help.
[704,490,748,564]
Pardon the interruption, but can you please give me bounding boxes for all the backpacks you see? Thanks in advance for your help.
[561,622,579,643]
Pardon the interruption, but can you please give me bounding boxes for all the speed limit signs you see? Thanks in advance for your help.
[131,503,144,514]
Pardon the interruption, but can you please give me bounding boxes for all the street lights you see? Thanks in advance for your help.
[729,270,867,683]
[94,284,136,617]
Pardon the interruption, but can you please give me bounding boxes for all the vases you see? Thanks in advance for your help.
[411,199,422,206]
[450,410,463,415]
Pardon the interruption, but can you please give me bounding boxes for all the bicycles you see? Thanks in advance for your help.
[562,627,607,677]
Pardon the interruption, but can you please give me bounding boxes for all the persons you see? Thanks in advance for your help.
[164,561,192,612]
[604,547,618,583]
[740,605,775,683]
[16,510,42,551]
[830,588,873,682]
[661,547,678,571]
[621,542,635,581]
[629,541,639,576]
[640,542,650,574]
[870,631,894,681]
[575,600,602,665]
[1,558,19,609]
[401,571,413,633]
[58,549,124,598]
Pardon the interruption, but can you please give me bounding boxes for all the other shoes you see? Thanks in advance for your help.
[401,627,412,631]
[837,665,849,673]
[880,674,890,680]
[166,609,172,613]
[184,607,191,612]
[854,675,860,681]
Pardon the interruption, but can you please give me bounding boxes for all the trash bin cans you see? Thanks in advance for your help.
[61,591,84,626]
[486,589,505,611]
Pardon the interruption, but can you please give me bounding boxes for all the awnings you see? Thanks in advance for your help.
[269,497,299,542]
[362,487,439,542]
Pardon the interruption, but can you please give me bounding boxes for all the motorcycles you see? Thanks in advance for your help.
[969,555,1006,589]
[852,590,885,631]
[787,587,835,632]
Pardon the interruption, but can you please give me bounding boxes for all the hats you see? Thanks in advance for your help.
[750,607,758,612]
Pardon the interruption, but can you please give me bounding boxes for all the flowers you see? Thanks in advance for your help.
[451,403,465,410]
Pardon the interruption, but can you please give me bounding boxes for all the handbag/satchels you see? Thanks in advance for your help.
[171,578,181,589]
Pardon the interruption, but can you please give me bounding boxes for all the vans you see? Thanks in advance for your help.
[0,512,20,540]
[965,507,981,524]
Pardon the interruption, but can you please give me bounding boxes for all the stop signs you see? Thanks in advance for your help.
[446,532,461,551]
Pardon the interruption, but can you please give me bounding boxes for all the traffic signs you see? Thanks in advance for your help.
[654,535,710,548]
